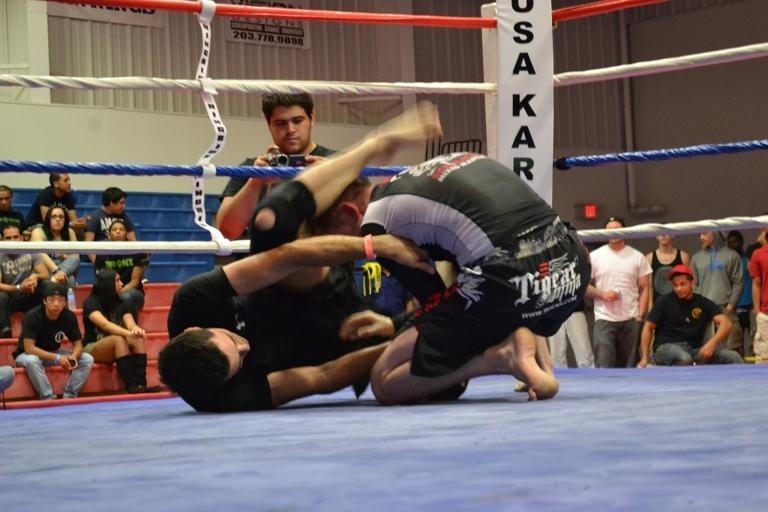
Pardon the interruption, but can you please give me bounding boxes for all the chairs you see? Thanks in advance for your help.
[643,320,715,367]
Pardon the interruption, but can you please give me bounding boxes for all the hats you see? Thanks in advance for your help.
[604,216,625,228]
[665,265,696,276]
[44,282,67,299]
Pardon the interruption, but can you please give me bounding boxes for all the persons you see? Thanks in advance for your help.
[1,174,152,399]
[157,104,446,412]
[217,95,338,263]
[549,215,768,369]
[306,152,593,406]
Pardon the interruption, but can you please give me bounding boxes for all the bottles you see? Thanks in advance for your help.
[67,287,76,311]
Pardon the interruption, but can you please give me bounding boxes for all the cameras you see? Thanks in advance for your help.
[270,150,310,167]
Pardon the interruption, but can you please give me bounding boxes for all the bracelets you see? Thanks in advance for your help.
[364,234,377,260]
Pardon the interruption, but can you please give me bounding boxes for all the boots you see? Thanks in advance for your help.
[115,353,161,393]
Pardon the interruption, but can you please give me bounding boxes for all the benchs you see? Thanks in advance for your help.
[0,187,226,408]
